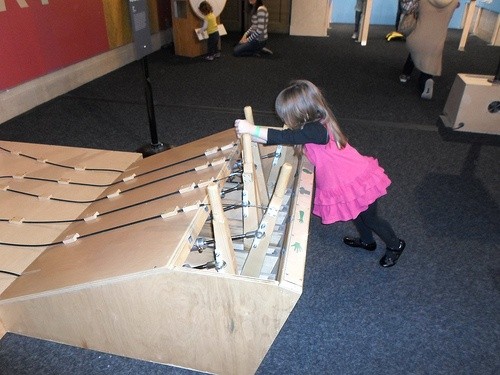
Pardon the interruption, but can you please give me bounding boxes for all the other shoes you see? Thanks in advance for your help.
[262,47,273,55]
[206,56,214,61]
[399,74,408,83]
[420,78,434,100]
[352,32,358,40]
[213,53,220,57]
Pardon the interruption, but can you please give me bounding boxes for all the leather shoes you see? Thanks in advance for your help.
[380,239,406,268]
[342,235,378,252]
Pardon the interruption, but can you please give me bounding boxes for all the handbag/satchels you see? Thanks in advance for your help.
[399,9,416,35]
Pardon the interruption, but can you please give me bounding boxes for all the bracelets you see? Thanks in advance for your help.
[254,126,261,136]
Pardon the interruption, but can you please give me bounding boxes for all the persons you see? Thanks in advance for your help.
[235,79,406,268]
[199,1,220,60]
[233,0,272,57]
[400,0,459,100]
[352,0,363,39]
